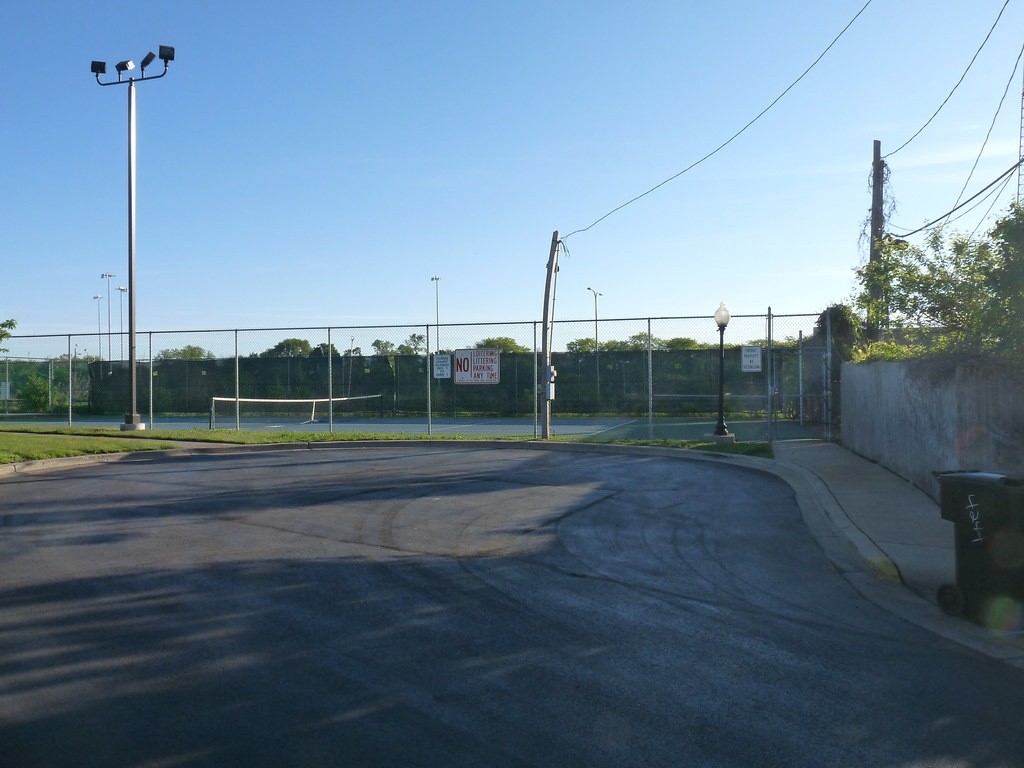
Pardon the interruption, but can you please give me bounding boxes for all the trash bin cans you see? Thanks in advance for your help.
[930,469,1024,630]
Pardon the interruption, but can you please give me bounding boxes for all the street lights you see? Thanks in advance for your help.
[431,275,443,353]
[713,302,733,435]
[587,287,604,397]
[100,272,116,376]
[92,295,105,384]
[114,285,128,378]
[87,41,178,433]
